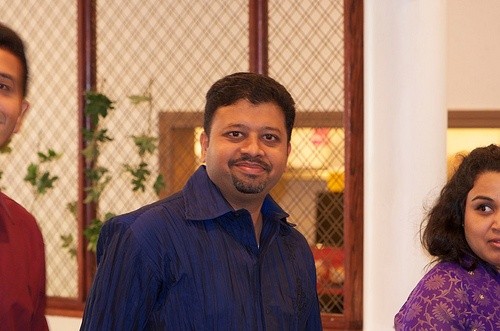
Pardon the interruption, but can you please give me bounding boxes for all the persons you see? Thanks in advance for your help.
[392,144,500,331]
[78,72,325,331]
[0,23,50,331]
